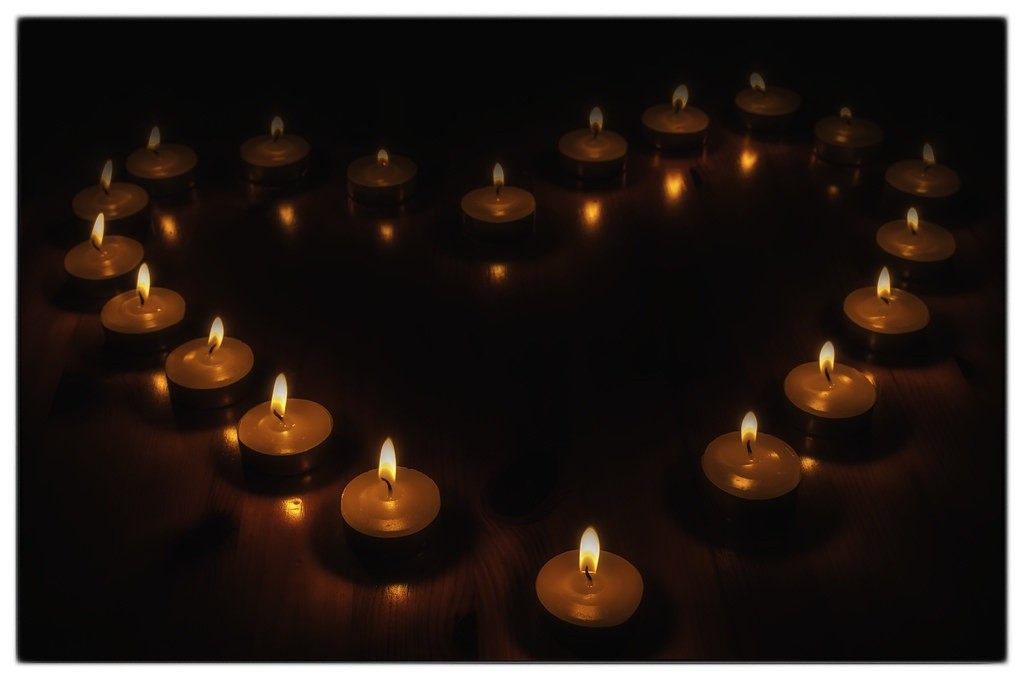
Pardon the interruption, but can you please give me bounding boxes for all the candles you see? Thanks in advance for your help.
[164,314,256,410]
[241,114,311,182]
[783,341,877,439]
[237,373,335,477]
[841,265,931,353]
[340,437,444,559]
[643,83,710,151]
[346,147,418,204]
[558,105,627,179]
[875,207,956,281]
[812,107,884,167]
[64,210,145,296]
[126,125,197,195]
[461,162,536,240]
[734,73,802,132]
[536,525,646,640]
[72,160,150,238]
[700,409,803,516]
[100,263,189,350]
[882,143,960,213]
